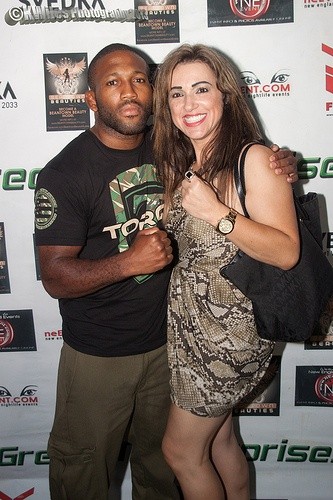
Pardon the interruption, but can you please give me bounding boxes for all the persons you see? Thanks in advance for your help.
[34,43,300,500]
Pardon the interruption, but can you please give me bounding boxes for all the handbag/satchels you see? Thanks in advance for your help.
[219,139,333,343]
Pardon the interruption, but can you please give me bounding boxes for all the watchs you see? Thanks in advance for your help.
[215,208,238,236]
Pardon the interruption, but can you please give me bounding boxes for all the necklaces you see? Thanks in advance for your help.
[190,160,196,172]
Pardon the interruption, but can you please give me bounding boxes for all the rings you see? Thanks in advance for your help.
[185,171,194,180]
